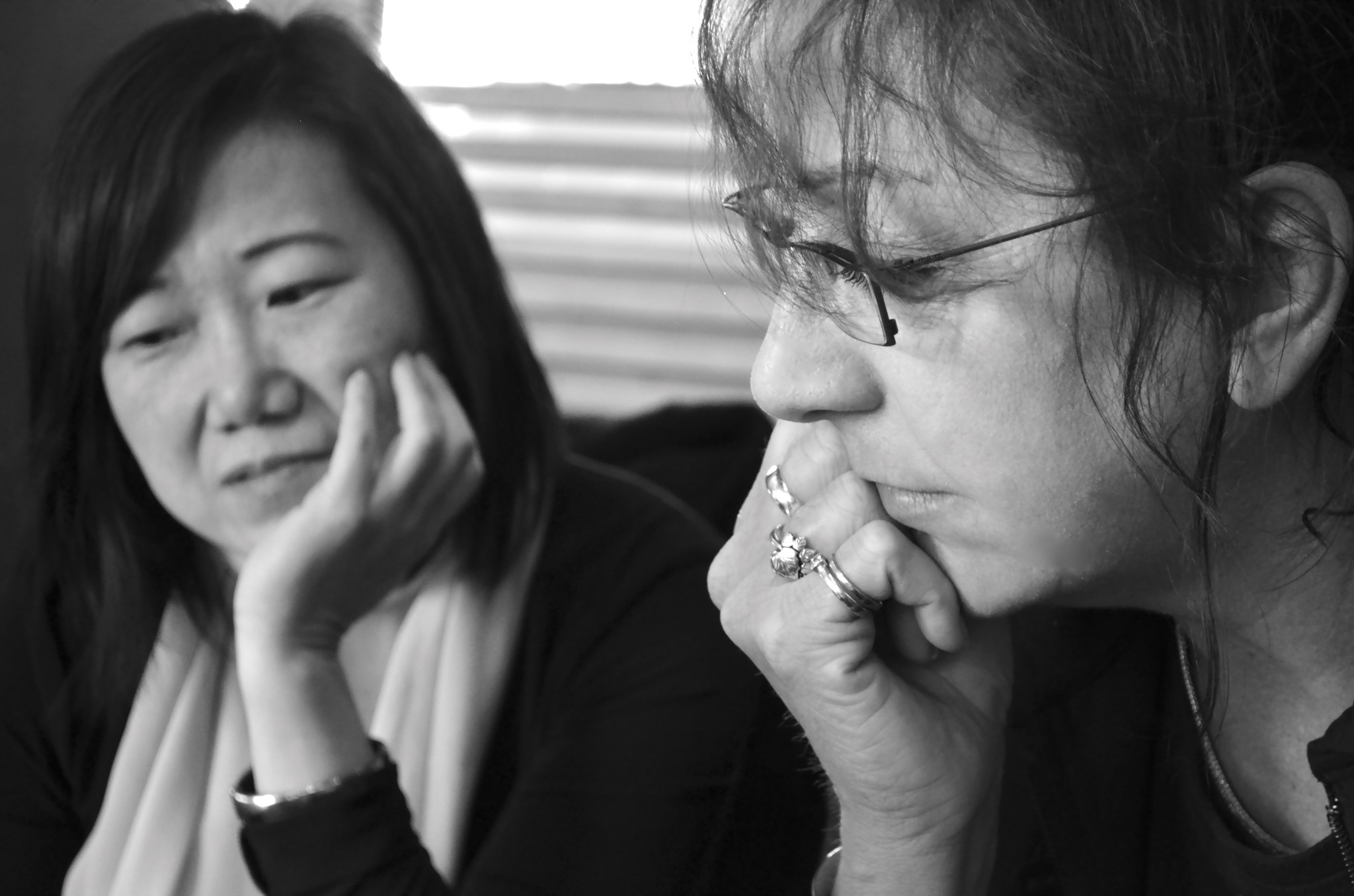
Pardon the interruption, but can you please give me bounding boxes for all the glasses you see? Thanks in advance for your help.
[721,172,1232,345]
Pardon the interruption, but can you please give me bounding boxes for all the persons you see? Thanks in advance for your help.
[705,0,1353,896]
[1,8,837,896]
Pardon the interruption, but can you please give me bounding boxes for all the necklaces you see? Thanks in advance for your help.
[1169,622,1308,857]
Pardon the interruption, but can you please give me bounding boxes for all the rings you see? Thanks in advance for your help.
[811,553,883,618]
[768,524,816,580]
[764,464,803,518]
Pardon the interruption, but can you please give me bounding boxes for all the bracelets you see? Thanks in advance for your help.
[229,738,392,820]
